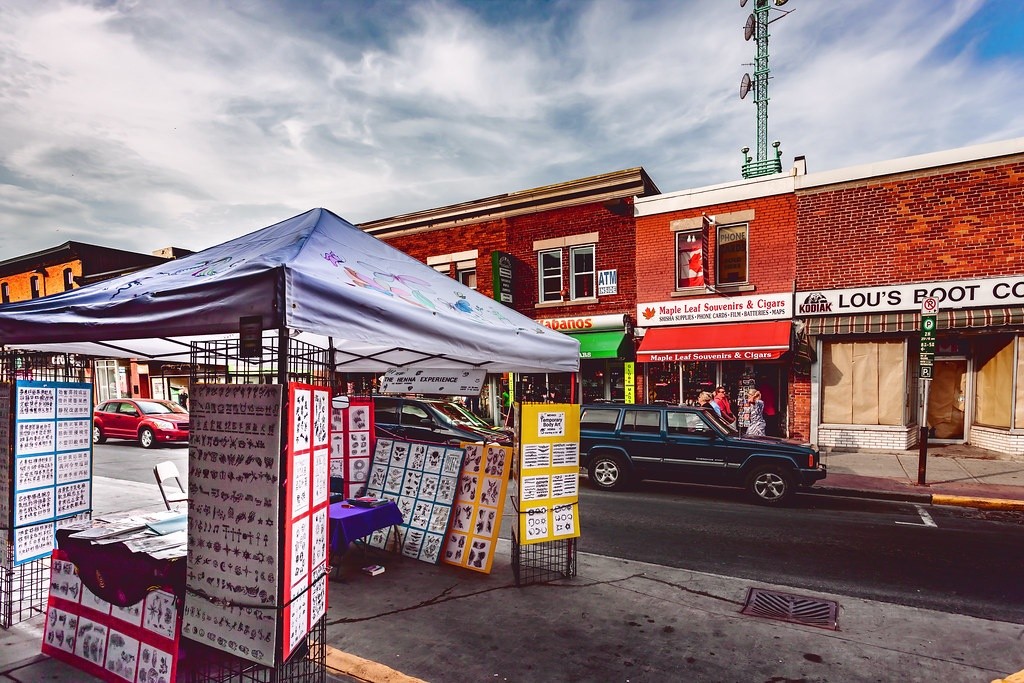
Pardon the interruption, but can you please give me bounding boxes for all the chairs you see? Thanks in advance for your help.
[152,461,188,510]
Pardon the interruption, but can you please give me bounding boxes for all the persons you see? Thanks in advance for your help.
[500,386,510,415]
[746,389,767,436]
[178,389,189,410]
[697,386,737,430]
[546,389,563,403]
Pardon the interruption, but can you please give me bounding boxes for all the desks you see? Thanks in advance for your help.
[329,497,403,577]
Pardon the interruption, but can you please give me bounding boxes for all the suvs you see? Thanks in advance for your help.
[372,393,514,448]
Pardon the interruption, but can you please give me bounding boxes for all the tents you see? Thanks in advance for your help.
[0,205,587,683]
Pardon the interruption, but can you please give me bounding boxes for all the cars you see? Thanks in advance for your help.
[93,398,189,449]
[579,403,827,507]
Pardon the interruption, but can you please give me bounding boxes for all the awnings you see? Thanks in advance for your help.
[566,332,628,360]
[634,318,794,362]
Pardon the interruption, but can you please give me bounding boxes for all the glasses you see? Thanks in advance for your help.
[719,391,726,393]
